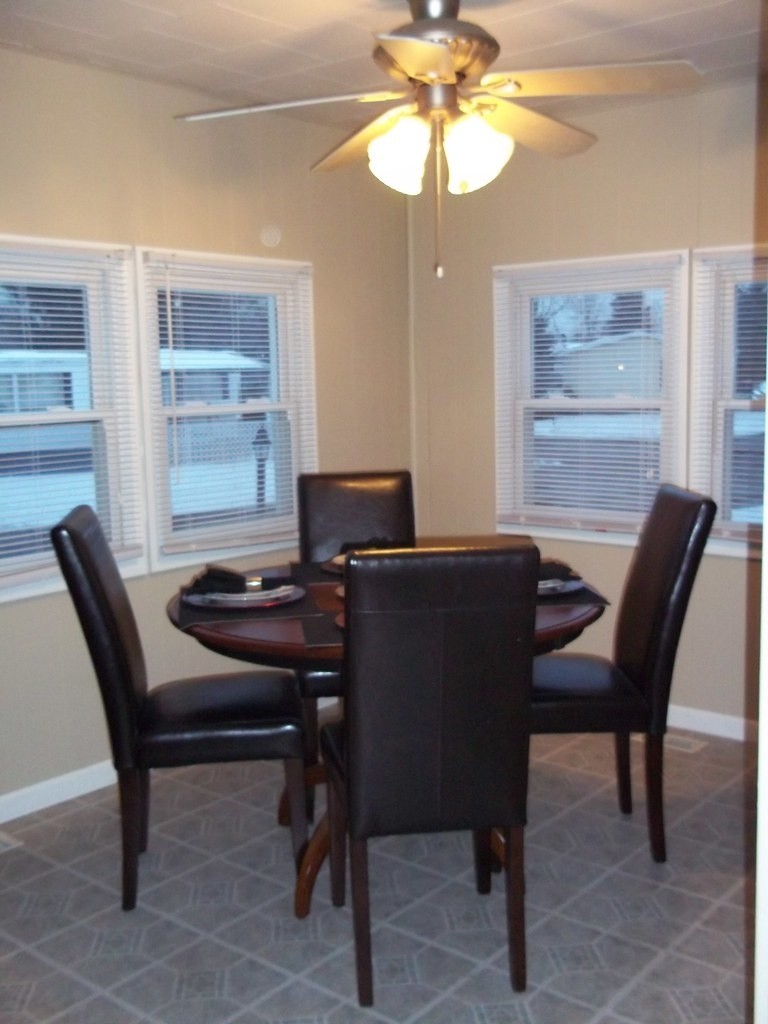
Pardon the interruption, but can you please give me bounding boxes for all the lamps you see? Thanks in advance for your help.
[367,0,515,194]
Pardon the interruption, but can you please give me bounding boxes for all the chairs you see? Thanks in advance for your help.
[530,481,720,865]
[276,471,415,827]
[49,506,317,917]
[317,543,549,1008]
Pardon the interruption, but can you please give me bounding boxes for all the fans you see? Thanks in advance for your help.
[172,0,705,174]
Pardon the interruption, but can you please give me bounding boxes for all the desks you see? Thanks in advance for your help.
[162,553,606,920]
